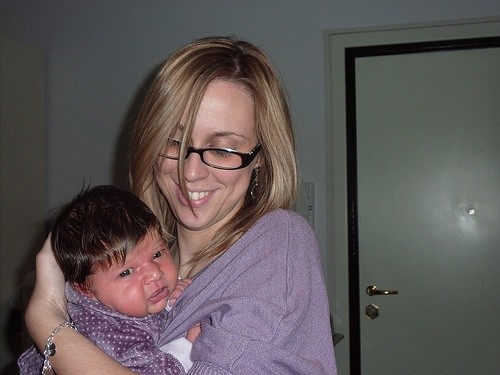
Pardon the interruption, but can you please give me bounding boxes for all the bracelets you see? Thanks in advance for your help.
[42,322,81,375]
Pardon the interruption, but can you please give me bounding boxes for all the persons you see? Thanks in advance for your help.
[17,185,203,375]
[24,36,337,375]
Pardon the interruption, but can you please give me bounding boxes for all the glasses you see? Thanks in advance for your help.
[159,138,262,170]
